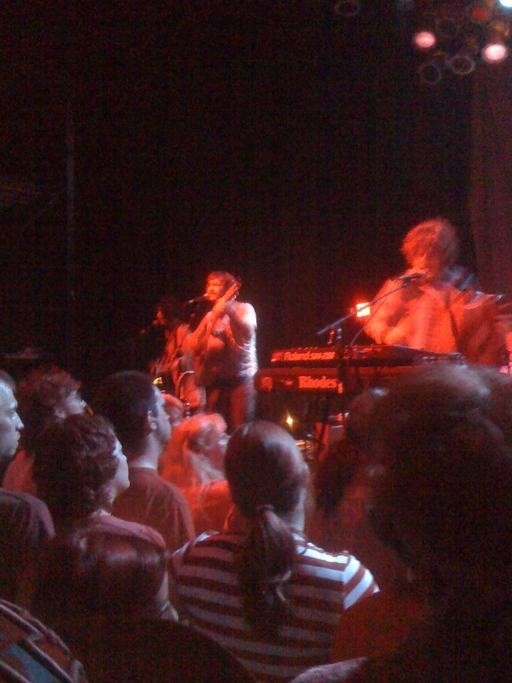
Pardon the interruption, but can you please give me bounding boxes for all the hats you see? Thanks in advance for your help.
[341,360,512,484]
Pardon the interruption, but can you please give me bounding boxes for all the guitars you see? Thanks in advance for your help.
[192,282,239,388]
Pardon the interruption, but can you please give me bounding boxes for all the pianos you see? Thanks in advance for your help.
[270,344,469,364]
[254,366,409,399]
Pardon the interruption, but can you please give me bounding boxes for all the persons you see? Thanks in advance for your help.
[0,347,512,680]
[146,296,209,409]
[357,216,472,363]
[176,267,260,434]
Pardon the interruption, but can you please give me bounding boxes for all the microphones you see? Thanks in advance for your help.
[135,319,161,336]
[184,292,211,307]
[396,270,423,283]
